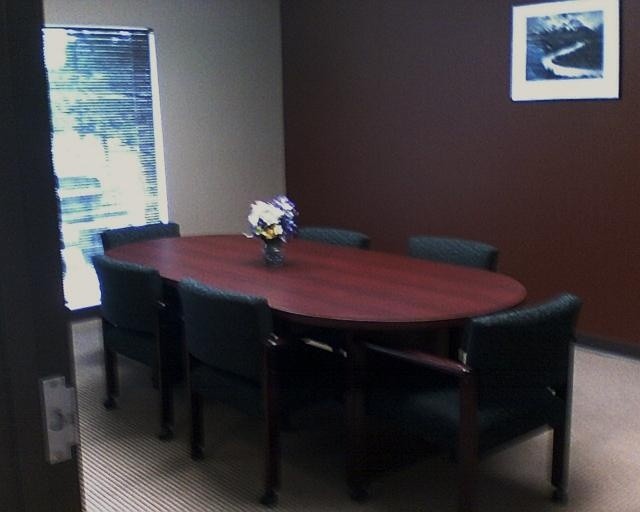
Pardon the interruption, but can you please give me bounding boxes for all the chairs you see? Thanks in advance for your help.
[176,277,289,508]
[98,220,183,255]
[90,253,187,445]
[349,291,581,512]
[294,225,373,252]
[406,234,507,283]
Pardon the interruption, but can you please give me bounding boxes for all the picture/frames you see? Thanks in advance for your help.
[507,2,622,107]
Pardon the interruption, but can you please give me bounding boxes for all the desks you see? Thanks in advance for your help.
[104,234,530,496]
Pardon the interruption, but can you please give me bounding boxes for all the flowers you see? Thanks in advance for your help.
[242,195,303,262]
